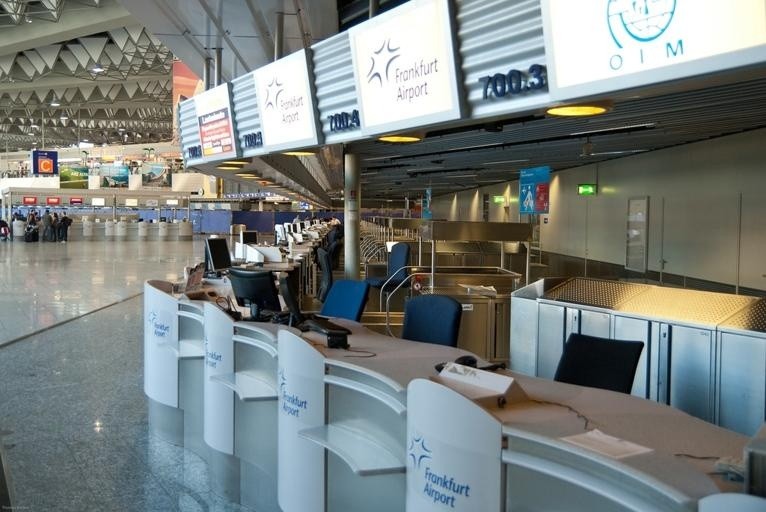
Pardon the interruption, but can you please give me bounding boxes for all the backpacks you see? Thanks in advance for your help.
[65,217,72,226]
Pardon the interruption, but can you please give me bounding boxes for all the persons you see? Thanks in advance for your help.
[12,209,72,243]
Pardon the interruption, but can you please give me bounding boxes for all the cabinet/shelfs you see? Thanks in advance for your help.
[537,302,760,435]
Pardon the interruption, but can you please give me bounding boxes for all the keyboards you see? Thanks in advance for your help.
[307,318,350,334]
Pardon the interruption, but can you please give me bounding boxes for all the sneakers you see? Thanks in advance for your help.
[42,238,66,243]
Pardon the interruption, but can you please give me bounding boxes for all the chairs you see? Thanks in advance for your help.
[315,223,463,348]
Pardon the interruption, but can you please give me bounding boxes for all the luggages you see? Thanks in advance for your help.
[25,229,39,242]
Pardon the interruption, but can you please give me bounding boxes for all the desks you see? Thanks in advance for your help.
[406,368,761,512]
[164,216,315,419]
[316,317,496,511]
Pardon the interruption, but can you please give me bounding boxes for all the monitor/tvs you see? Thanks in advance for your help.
[205,238,232,277]
[275,219,321,243]
[279,271,304,327]
[229,269,281,318]
[240,230,258,243]
